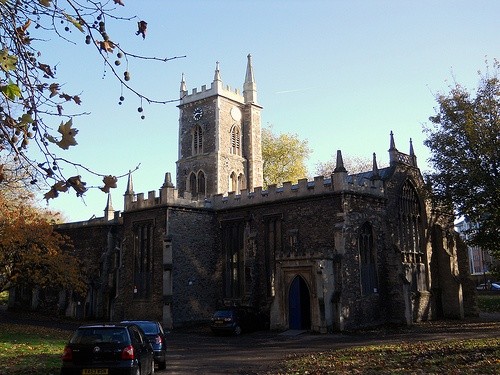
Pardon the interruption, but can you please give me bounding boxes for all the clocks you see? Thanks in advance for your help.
[192,107,203,120]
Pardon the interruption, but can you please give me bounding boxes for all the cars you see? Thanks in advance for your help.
[119,320,167,369]
[209,306,266,336]
[59,322,156,375]
